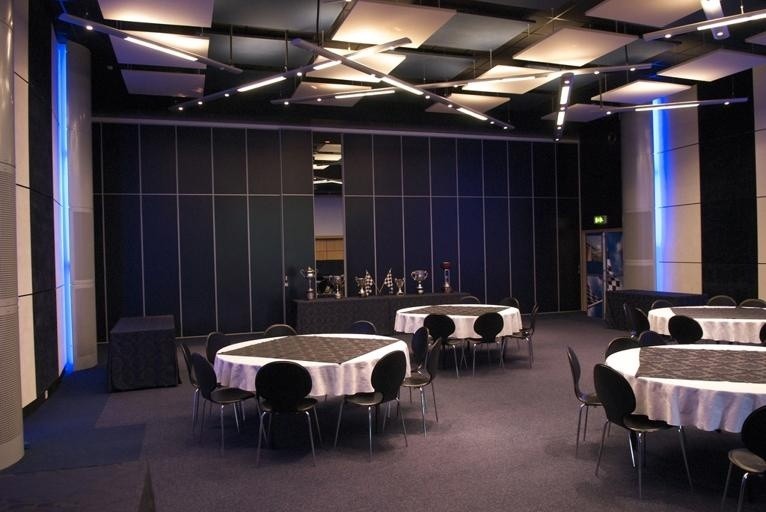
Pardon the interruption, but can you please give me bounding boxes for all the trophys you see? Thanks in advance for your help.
[300,264,320,300]
[395,277,405,295]
[328,273,346,299]
[316,275,335,295]
[410,270,428,294]
[353,274,366,296]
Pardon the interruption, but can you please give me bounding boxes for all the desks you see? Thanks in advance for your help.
[104,313,183,391]
[293,288,470,340]
[214,330,413,438]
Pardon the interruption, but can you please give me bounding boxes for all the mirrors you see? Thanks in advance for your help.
[311,130,349,299]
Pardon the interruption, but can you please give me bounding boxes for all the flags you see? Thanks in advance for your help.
[363,270,374,296]
[605,249,623,292]
[384,270,393,294]
[586,280,601,307]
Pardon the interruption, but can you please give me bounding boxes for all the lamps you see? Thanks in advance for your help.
[603,92,751,117]
[312,151,342,188]
[63,6,245,76]
[168,31,653,135]
[637,3,766,49]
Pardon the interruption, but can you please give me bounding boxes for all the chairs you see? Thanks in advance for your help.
[331,349,410,462]
[349,317,378,335]
[721,401,766,511]
[262,324,300,339]
[637,329,665,348]
[394,295,542,378]
[252,357,325,470]
[180,343,243,436]
[203,333,262,421]
[188,351,268,455]
[620,294,766,347]
[607,336,641,362]
[591,362,691,497]
[565,347,637,466]
